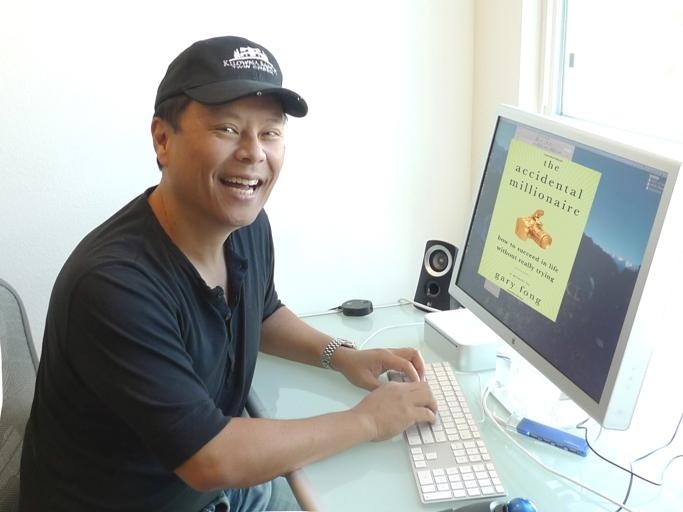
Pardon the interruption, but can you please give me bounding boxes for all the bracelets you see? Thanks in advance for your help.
[320,336,358,371]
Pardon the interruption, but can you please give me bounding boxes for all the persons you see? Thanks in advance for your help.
[16,32,438,512]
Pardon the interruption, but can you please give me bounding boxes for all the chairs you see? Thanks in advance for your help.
[1,277,38,512]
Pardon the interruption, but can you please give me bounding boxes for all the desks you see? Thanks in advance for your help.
[237,298,677,511]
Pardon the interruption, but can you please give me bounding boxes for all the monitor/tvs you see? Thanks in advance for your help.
[447,101,683,431]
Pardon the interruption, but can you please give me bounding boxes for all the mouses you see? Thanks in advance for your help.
[488,498,535,512]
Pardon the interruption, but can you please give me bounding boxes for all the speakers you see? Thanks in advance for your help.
[412,239,459,313]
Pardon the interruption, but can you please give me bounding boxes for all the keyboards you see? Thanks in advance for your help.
[387,360,508,505]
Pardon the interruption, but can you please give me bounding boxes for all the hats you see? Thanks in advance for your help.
[155,36,308,117]
